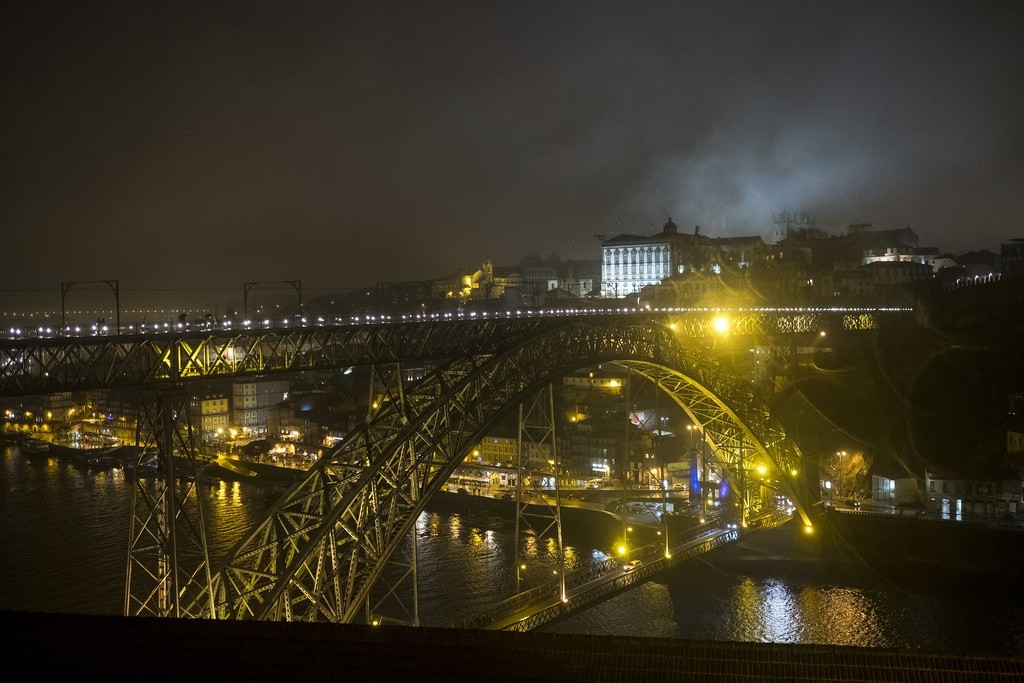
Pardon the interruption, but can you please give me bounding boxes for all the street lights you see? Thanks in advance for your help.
[837,451,846,497]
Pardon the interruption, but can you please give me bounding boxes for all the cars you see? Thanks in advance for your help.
[457,487,470,493]
[586,479,605,489]
[673,483,685,489]
[846,499,860,507]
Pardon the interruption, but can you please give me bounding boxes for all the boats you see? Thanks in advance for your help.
[22,437,49,455]
